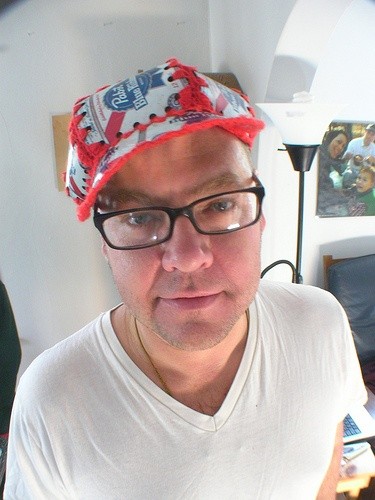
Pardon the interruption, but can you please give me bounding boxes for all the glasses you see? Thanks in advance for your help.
[93,176,266,250]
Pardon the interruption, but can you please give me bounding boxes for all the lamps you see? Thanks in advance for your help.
[255,102,345,283]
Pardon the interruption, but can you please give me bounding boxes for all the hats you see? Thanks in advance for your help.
[62,61,264,225]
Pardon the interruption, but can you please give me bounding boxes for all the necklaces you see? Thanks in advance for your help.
[131,308,251,400]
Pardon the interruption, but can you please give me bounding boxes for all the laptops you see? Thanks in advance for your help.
[342,406,375,444]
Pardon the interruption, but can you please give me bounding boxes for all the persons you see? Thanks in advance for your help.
[318,124,375,216]
[0,279,24,500]
[4,61,367,500]
